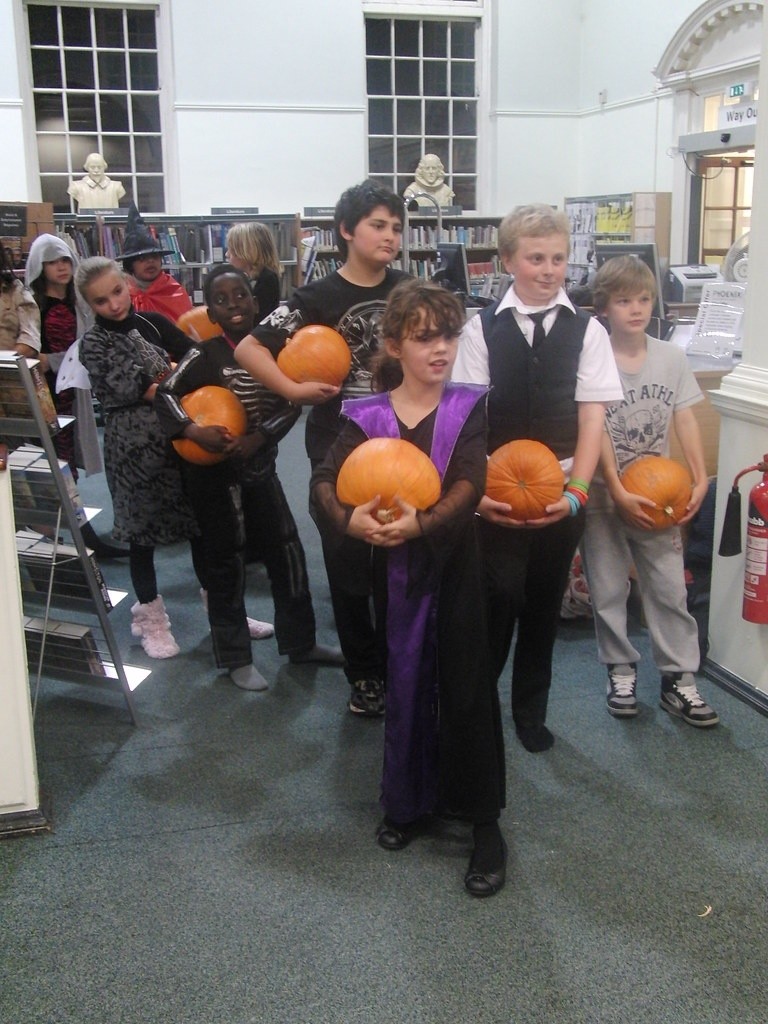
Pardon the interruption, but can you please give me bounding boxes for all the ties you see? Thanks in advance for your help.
[528,305,557,350]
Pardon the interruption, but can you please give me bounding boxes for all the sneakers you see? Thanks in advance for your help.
[608,663,639,715]
[659,675,720,727]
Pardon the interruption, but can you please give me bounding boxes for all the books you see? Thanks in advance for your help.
[0,350,62,436]
[23,615,106,678]
[14,526,114,614]
[55,222,230,307]
[8,442,87,526]
[265,222,345,302]
[386,223,514,298]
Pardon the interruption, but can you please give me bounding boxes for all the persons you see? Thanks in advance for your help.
[311,280,508,900]
[113,200,195,327]
[220,224,284,566]
[23,232,104,557]
[0,242,43,418]
[582,256,718,730]
[70,258,274,660]
[66,152,126,213]
[448,195,629,754]
[233,181,427,715]
[402,153,456,206]
[150,263,348,688]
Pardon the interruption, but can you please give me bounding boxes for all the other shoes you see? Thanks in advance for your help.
[464,838,508,896]
[375,815,416,850]
[349,699,385,715]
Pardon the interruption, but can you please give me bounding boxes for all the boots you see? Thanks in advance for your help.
[200,589,275,639]
[132,594,180,659]
[50,535,64,544]
[80,521,131,558]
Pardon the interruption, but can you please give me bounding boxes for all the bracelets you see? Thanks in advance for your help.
[562,478,590,517]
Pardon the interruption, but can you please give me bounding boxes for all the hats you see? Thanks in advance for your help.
[114,200,175,262]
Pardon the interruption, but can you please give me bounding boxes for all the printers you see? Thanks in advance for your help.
[667,264,726,304]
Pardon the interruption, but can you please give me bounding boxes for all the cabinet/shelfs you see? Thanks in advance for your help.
[0,192,672,310]
[0,355,153,728]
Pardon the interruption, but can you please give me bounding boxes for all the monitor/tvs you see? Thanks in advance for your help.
[594,241,666,321]
[431,242,471,296]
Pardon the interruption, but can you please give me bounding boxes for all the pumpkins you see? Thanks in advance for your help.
[277,325,352,387]
[483,440,566,522]
[170,385,247,466]
[618,456,691,531]
[335,437,441,524]
[176,305,223,341]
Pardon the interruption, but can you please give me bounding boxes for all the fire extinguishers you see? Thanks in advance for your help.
[719,454,768,625]
